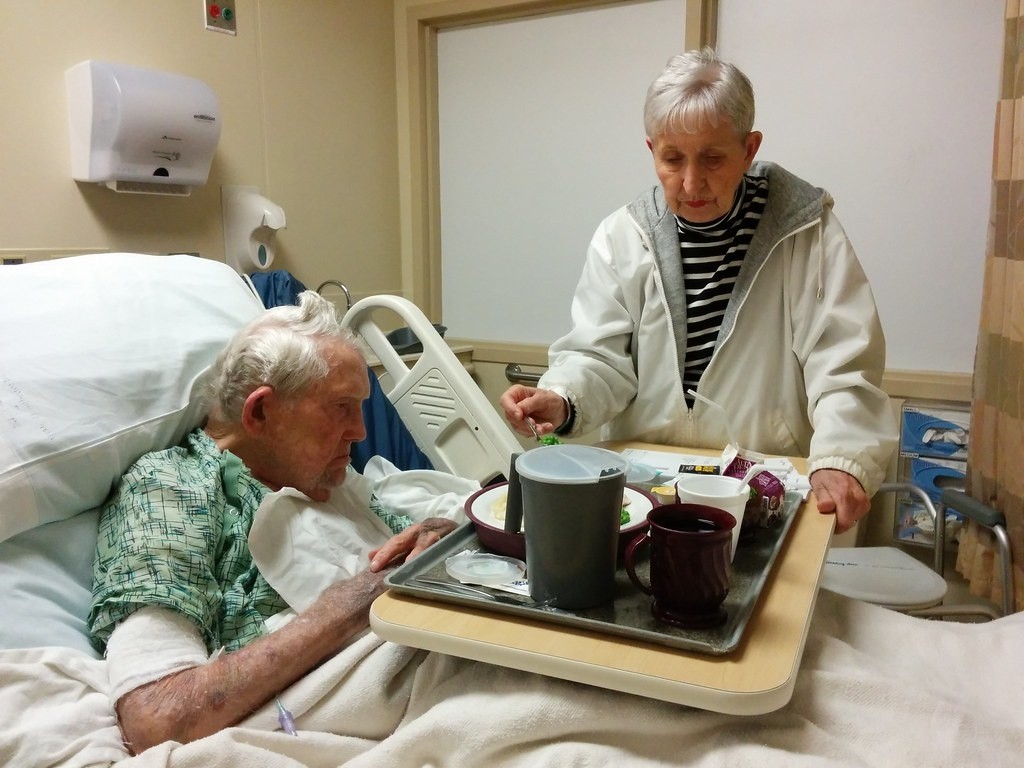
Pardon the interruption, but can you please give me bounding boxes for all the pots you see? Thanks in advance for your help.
[464,480,660,561]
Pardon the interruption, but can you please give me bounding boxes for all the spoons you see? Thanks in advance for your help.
[522,413,565,446]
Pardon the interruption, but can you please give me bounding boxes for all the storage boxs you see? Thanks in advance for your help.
[891,398,971,551]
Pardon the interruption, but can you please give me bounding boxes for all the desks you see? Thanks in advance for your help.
[372,441,839,716]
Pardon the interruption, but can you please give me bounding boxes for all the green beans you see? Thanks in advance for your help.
[621,508,630,524]
[541,436,563,445]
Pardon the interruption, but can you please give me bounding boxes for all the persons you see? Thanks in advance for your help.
[86,291,463,753]
[498,51,894,534]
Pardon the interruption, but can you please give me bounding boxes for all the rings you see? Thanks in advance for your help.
[854,520,857,524]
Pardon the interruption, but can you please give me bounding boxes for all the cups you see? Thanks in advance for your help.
[625,504,738,629]
[677,475,751,564]
[516,443,631,611]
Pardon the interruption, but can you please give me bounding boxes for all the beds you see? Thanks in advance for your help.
[2,290,1024,764]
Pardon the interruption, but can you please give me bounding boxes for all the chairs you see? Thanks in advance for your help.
[818,479,1017,624]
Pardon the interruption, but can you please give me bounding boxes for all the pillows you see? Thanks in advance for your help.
[0,252,265,544]
